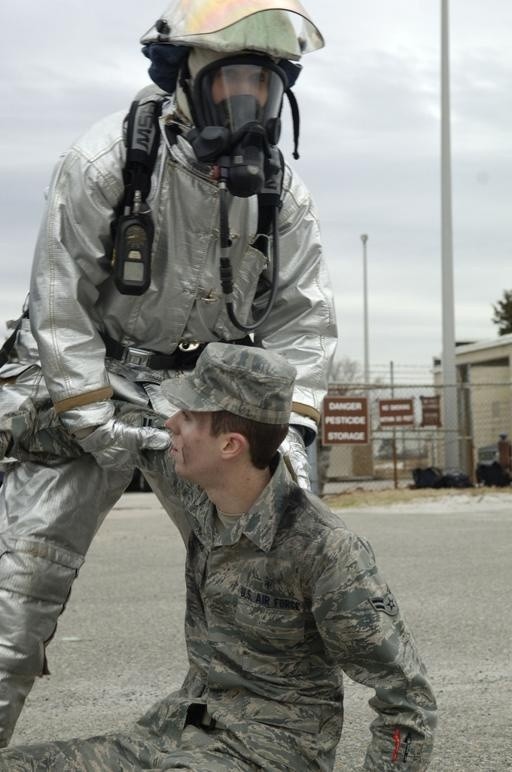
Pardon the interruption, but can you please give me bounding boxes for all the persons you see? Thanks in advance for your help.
[1,0,339,747]
[0,342,438,772]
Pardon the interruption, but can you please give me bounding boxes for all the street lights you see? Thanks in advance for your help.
[359,233,371,406]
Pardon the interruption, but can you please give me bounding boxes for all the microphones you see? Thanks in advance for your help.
[285,90,299,160]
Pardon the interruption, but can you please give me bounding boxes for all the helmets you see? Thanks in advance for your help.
[138,0,326,59]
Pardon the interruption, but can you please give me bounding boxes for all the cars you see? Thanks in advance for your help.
[474,440,511,468]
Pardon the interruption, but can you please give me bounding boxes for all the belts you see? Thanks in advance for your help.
[186,702,232,731]
[99,329,258,371]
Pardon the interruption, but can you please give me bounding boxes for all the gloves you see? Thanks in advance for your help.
[76,419,167,474]
[274,430,316,493]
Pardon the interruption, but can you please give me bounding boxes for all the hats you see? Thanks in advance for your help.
[158,342,296,427]
